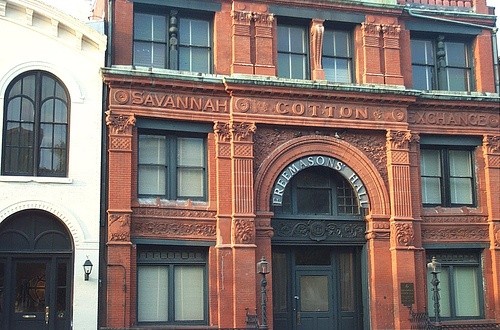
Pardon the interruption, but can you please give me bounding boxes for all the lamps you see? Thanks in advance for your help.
[82,259,93,281]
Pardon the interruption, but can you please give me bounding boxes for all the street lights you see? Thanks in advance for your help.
[257,256,271,330]
[427,257,443,330]
